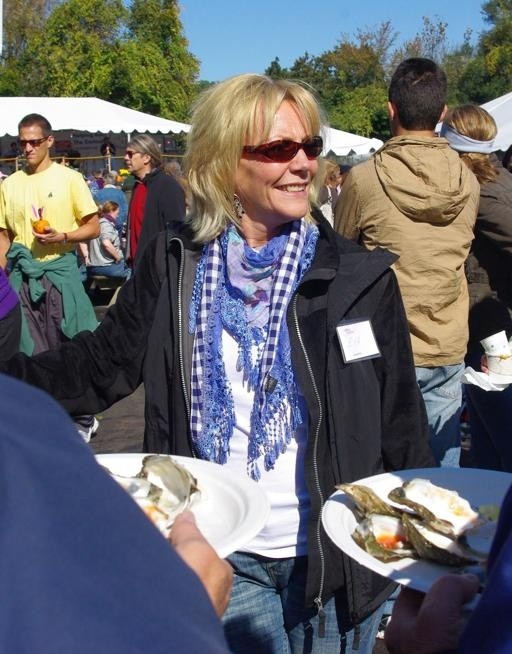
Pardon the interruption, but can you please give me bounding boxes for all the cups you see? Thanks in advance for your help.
[480,330,512,355]
[485,353,512,384]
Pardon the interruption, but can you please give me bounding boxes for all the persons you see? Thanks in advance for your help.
[0,375,234,653]
[438,102,512,474]
[384,483,511,654]
[1,74,441,653]
[315,159,342,228]
[80,168,132,281]
[126,132,185,274]
[0,112,99,442]
[315,57,480,469]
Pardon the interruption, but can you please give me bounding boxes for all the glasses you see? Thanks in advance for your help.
[241,136,322,162]
[19,138,48,146]
[127,151,139,158]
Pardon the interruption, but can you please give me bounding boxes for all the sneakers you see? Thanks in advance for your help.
[78,417,99,444]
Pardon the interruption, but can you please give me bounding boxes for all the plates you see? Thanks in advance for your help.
[321,468,512,611]
[94,453,269,559]
